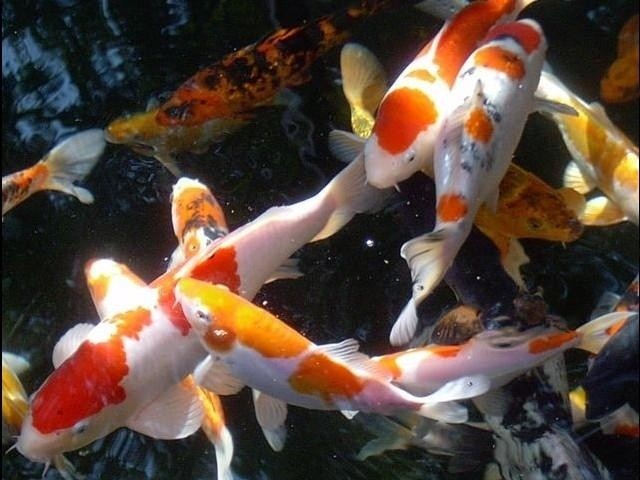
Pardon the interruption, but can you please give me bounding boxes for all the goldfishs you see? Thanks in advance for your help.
[0,0,640,480]
